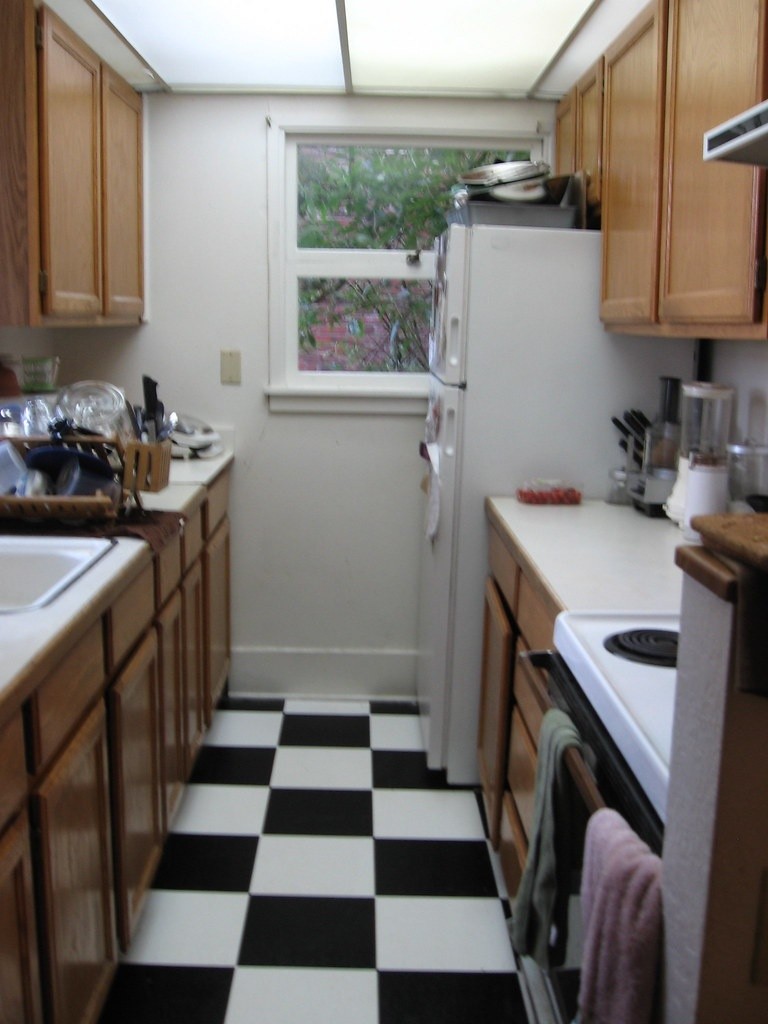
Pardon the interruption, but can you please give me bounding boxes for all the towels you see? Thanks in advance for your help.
[506,705,599,979]
[572,808,669,1024]
[1,503,194,555]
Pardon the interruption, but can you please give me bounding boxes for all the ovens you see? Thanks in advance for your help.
[516,648,664,1024]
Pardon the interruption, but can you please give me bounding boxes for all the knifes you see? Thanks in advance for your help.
[123,375,180,492]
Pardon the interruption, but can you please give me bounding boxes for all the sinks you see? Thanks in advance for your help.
[0,528,119,618]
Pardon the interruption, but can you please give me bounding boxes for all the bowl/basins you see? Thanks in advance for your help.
[444,159,576,224]
[55,379,130,446]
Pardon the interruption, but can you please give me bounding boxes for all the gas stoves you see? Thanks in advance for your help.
[604,624,680,669]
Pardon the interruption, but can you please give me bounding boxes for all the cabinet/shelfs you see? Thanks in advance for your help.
[1,614,119,1024]
[601,0,767,340]
[556,55,602,219]
[1,0,147,327]
[179,467,232,774]
[476,523,560,908]
[109,526,188,955]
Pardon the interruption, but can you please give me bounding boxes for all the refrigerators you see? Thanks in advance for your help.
[421,223,694,785]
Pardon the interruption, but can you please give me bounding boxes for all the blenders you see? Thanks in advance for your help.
[662,380,734,541]
[629,376,682,518]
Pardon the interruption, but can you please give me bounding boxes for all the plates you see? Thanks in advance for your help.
[25,446,115,494]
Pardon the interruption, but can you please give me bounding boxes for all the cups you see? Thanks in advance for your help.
[21,354,60,392]
